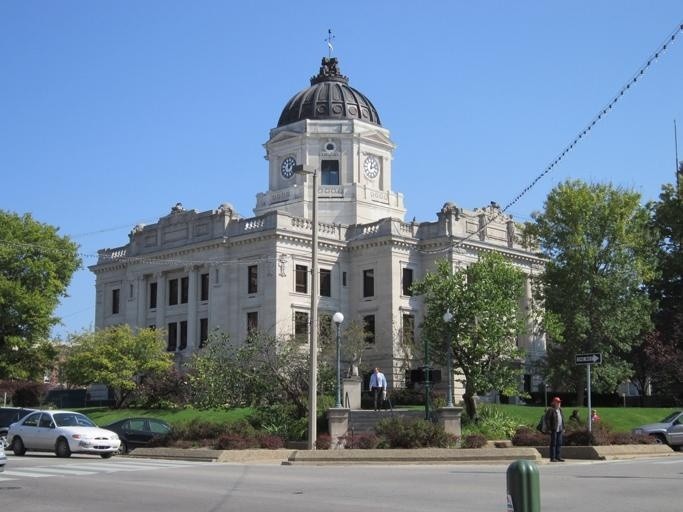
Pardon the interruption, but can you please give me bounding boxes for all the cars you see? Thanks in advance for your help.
[95,414,183,457]
[628,410,682,452]
[0,404,51,451]
[6,409,122,459]
[0,441,7,473]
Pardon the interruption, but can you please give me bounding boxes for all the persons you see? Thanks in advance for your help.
[545,397,566,463]
[570,409,582,429]
[588,409,600,424]
[541,408,550,434]
[369,367,387,411]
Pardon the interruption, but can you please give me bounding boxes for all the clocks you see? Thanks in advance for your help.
[280,156,296,179]
[362,155,379,179]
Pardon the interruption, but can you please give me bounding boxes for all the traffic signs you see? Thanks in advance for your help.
[574,352,604,367]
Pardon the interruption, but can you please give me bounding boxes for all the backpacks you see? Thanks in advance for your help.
[536,414,547,434]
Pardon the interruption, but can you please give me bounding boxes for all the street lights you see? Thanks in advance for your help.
[331,312,345,408]
[290,160,321,452]
[440,310,460,406]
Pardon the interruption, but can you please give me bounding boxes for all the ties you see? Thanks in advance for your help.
[376,374,379,387]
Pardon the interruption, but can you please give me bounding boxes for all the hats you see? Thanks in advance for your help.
[553,397,561,403]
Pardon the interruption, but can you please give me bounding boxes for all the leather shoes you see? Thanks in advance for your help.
[551,458,558,462]
[556,458,565,462]
